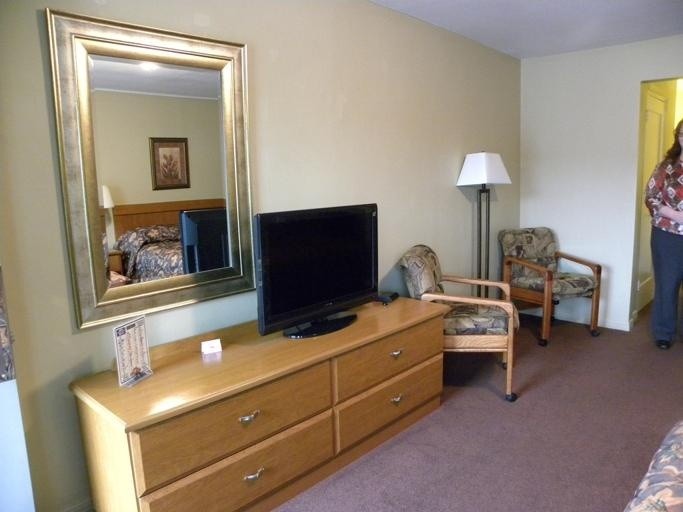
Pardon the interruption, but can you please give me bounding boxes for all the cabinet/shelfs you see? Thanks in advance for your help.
[68,298,451,512]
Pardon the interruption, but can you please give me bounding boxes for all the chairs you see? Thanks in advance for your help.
[622,419,683,512]
[397,243,519,400]
[498,227,602,346]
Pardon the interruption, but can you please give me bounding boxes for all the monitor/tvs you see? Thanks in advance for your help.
[180,206,229,273]
[254,202,378,338]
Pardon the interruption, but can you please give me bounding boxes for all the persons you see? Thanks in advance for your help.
[644,119,683,350]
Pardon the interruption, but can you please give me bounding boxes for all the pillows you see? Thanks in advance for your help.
[117,224,180,248]
[108,270,133,289]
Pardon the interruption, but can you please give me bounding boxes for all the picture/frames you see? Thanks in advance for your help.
[148,139,191,189]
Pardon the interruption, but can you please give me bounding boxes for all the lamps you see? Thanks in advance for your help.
[456,152,513,297]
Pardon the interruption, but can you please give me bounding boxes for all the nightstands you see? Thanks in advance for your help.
[107,249,124,276]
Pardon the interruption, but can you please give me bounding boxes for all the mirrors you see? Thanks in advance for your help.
[44,6,257,330]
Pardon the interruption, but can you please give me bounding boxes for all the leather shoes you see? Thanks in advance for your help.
[658,340,670,349]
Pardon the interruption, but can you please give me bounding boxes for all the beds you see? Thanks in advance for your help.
[98,197,226,285]
